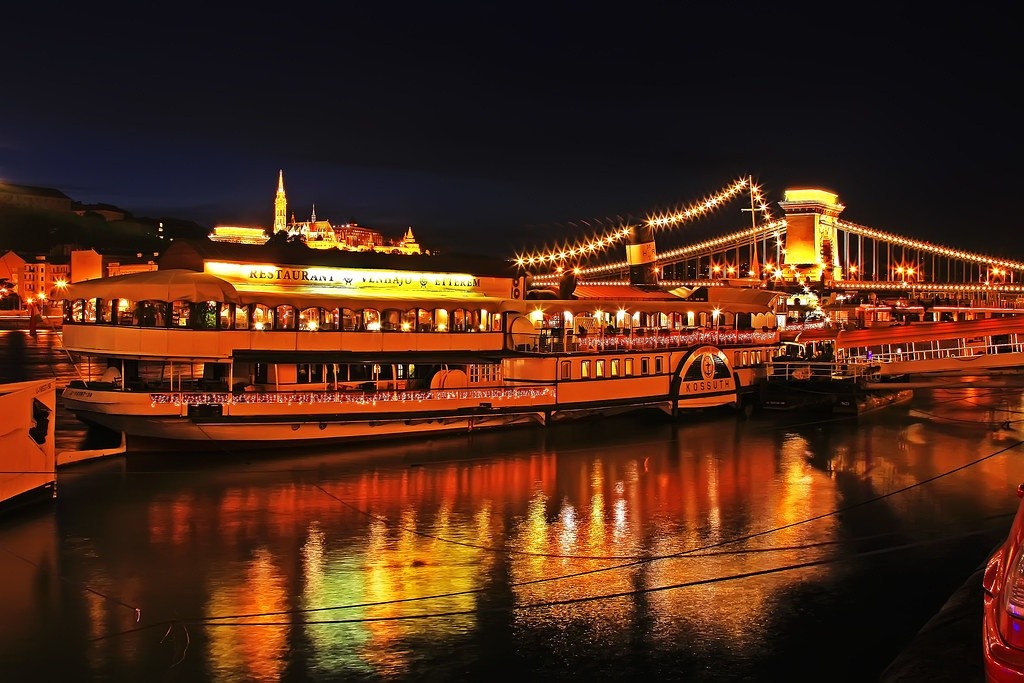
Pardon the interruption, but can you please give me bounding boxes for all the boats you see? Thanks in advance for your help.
[56,264,885,443]
[727,342,917,437]
[0,378,64,505]
